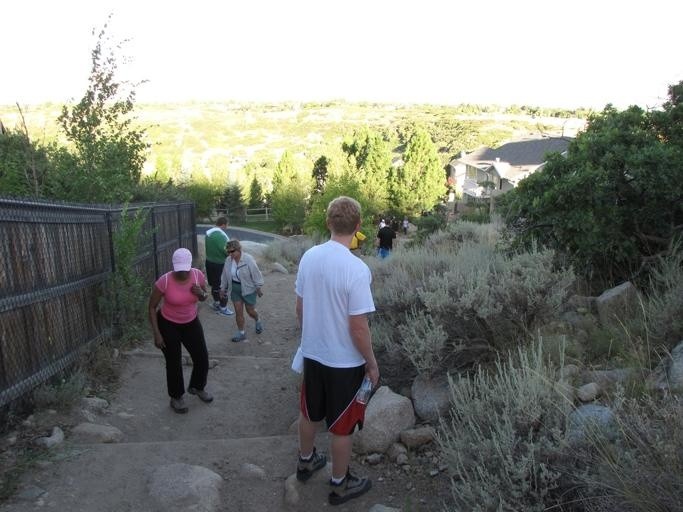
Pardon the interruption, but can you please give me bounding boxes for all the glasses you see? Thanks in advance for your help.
[227,250,235,253]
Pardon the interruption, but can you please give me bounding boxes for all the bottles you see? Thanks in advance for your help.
[356,373,373,405]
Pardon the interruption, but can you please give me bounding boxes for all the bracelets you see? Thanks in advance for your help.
[200,290,204,298]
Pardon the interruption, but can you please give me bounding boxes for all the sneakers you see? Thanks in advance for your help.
[210,302,233,315]
[232,330,246,342]
[296,446,326,479]
[255,319,262,334]
[187,387,212,402]
[329,472,371,505]
[170,397,188,413]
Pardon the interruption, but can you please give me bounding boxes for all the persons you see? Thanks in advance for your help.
[290,195,381,505]
[146,247,215,412]
[219,239,266,343]
[376,217,395,259]
[348,231,367,257]
[377,214,410,236]
[204,216,235,318]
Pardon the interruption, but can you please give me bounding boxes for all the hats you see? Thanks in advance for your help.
[171,247,192,272]
